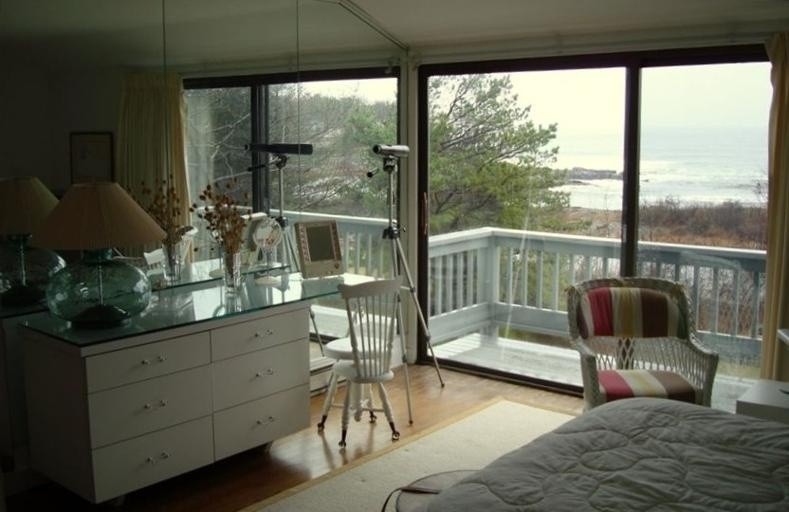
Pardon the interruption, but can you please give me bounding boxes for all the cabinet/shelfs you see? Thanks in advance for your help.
[16,275,315,504]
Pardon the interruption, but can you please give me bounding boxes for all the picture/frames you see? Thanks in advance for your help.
[293,219,344,278]
[70,131,114,185]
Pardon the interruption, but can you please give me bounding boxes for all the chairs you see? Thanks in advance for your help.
[567,275,719,414]
[317,274,404,448]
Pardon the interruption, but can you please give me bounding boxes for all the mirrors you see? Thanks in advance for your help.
[0,0,408,399]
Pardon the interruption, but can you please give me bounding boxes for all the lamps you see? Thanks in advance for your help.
[24,177,167,331]
[0,175,67,307]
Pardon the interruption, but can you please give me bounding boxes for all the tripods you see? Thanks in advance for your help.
[255,158,327,359]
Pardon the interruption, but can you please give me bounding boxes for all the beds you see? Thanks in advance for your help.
[426,396,789,512]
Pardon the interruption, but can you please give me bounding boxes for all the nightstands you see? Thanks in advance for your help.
[736,378,789,421]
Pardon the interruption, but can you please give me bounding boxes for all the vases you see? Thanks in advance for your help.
[162,243,181,284]
[223,252,242,292]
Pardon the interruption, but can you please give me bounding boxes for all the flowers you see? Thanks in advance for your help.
[123,172,194,270]
[188,174,253,281]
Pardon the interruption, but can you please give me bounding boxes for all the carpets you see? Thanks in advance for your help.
[236,397,580,512]
